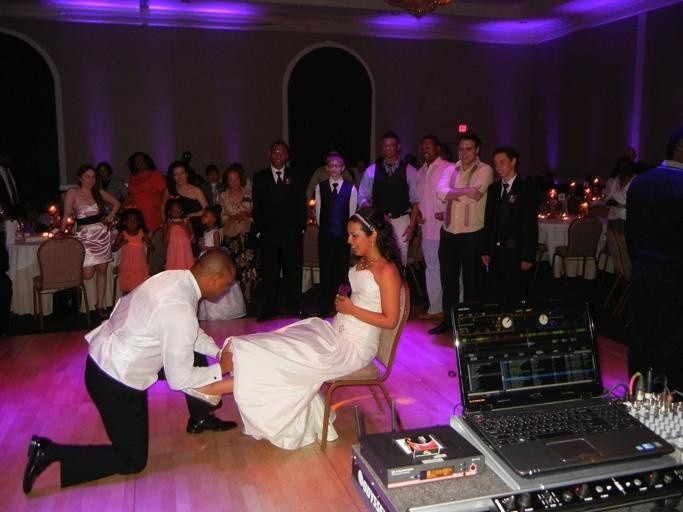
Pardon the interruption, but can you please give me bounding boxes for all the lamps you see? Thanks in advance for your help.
[383,1,455,20]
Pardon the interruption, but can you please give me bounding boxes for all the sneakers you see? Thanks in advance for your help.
[94,303,110,320]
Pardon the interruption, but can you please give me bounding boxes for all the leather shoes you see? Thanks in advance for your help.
[427,317,452,335]
[185,413,238,436]
[20,434,51,497]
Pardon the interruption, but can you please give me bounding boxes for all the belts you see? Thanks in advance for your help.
[73,215,102,225]
[385,212,411,218]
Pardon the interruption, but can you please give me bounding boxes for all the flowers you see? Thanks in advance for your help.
[337,282,351,295]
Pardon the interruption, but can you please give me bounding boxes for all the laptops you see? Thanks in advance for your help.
[451,297,675,478]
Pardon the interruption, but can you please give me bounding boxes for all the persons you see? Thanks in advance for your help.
[180,206,407,450]
[21,248,240,495]
[623,146,638,162]
[624,125,683,402]
[604,156,638,279]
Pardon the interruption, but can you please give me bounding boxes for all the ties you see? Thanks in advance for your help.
[4,169,18,204]
[500,182,511,212]
[386,164,395,182]
[331,182,339,199]
[275,171,281,186]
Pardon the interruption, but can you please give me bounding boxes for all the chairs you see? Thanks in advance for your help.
[535,217,634,327]
[31,236,92,335]
[300,221,319,287]
[110,225,167,305]
[315,275,411,452]
